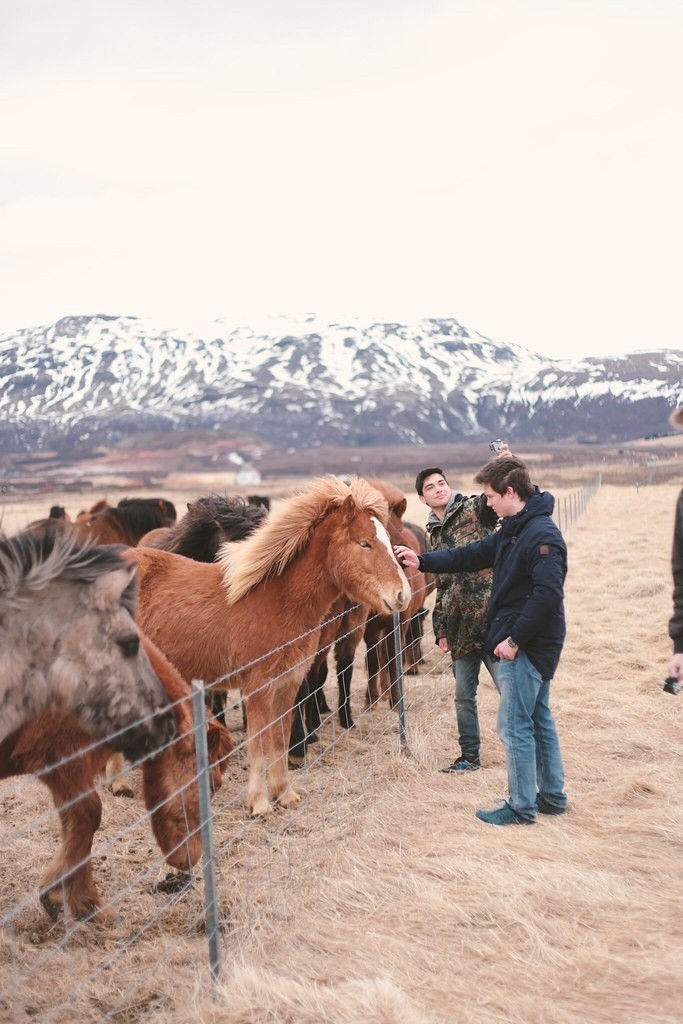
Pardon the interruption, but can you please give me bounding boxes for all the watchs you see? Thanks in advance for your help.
[507,637,516,648]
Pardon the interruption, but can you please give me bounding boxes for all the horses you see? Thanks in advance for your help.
[0,474,436,927]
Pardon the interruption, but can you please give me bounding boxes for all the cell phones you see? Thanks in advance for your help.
[489,441,505,453]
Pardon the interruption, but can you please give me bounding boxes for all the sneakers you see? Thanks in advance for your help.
[536,793,566,815]
[441,757,479,773]
[474,798,535,825]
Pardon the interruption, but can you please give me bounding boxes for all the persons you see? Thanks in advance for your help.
[667,407,683,683]
[394,459,568,826]
[414,439,516,774]
